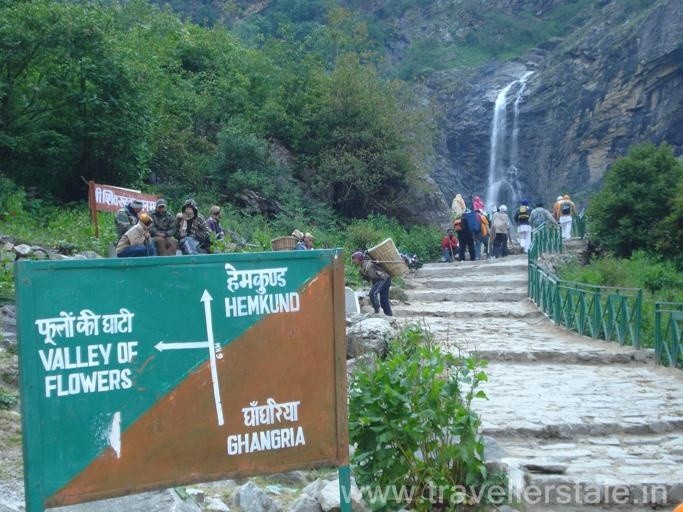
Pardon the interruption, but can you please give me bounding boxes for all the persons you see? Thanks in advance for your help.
[114,198,226,257]
[441,189,579,261]
[350,251,394,317]
[295,232,316,251]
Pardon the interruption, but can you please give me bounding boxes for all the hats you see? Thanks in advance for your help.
[131,199,167,225]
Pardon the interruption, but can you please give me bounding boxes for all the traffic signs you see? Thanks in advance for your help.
[13,249,350,512]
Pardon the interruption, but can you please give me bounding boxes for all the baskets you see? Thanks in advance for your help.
[271,236,298,251]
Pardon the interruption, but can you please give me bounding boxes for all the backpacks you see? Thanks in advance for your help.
[563,201,570,215]
[518,206,529,220]
